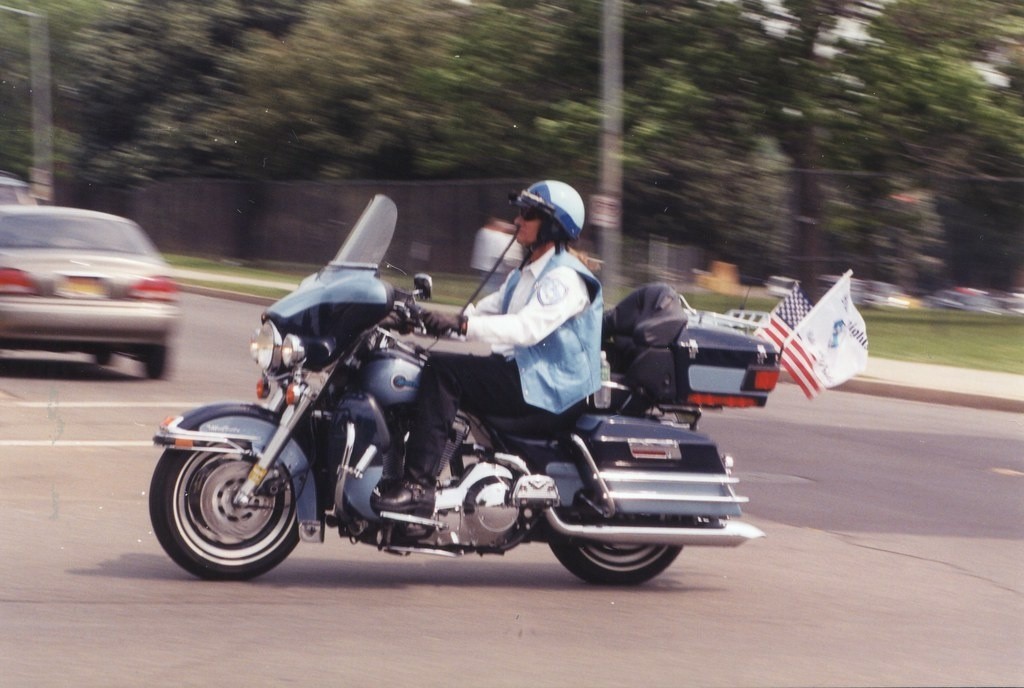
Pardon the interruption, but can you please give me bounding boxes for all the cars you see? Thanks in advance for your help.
[767,276,1024,317]
[0,205,179,377]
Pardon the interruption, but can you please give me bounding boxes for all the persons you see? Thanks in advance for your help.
[372,180,602,513]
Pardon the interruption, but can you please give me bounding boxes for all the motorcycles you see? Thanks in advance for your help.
[147,193,781,585]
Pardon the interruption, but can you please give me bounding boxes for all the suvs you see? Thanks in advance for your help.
[0,170,38,206]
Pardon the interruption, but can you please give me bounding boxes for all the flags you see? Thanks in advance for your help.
[798,274,868,388]
[753,282,827,400]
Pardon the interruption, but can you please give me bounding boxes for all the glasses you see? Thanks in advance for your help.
[520,207,549,220]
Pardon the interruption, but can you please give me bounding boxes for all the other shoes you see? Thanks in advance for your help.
[374,478,435,518]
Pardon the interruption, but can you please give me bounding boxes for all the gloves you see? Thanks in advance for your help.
[423,311,466,336]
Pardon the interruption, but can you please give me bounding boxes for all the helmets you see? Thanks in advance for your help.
[509,180,585,240]
